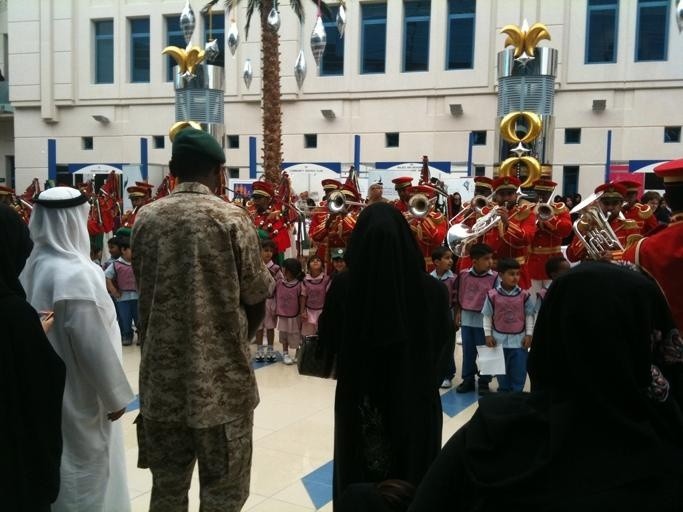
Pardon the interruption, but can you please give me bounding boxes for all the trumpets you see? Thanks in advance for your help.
[408,195,438,219]
[327,190,368,213]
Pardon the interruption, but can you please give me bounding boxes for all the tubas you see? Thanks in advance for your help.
[569,191,624,262]
[446,202,510,258]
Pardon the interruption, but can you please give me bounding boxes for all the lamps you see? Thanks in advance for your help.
[448,103,464,116]
[92,112,111,124]
[320,109,337,120]
[592,98,606,111]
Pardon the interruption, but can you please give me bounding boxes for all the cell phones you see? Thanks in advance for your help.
[38,312,53,321]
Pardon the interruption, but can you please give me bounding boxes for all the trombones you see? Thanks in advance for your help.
[448,191,496,226]
[535,188,555,226]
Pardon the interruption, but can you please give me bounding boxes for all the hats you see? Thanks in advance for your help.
[594,158,683,203]
[392,177,437,201]
[126,180,154,200]
[474,176,558,196]
[0,186,15,196]
[171,128,226,166]
[250,181,274,202]
[321,179,358,200]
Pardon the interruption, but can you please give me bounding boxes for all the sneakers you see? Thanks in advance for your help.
[438,376,492,395]
[253,350,301,364]
[123,331,141,346]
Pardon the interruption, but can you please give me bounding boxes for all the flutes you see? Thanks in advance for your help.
[125,207,137,226]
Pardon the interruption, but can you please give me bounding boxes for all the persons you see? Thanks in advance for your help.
[298,201,456,512]
[1,159,683,449]
[19,186,137,512]
[335,258,683,512]
[1,204,66,512]
[130,127,277,512]
